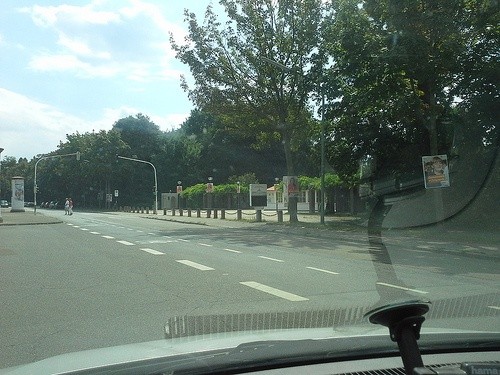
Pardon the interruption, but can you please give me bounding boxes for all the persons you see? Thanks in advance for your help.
[64,198,69,215]
[424,156,449,188]
[67,198,73,216]
